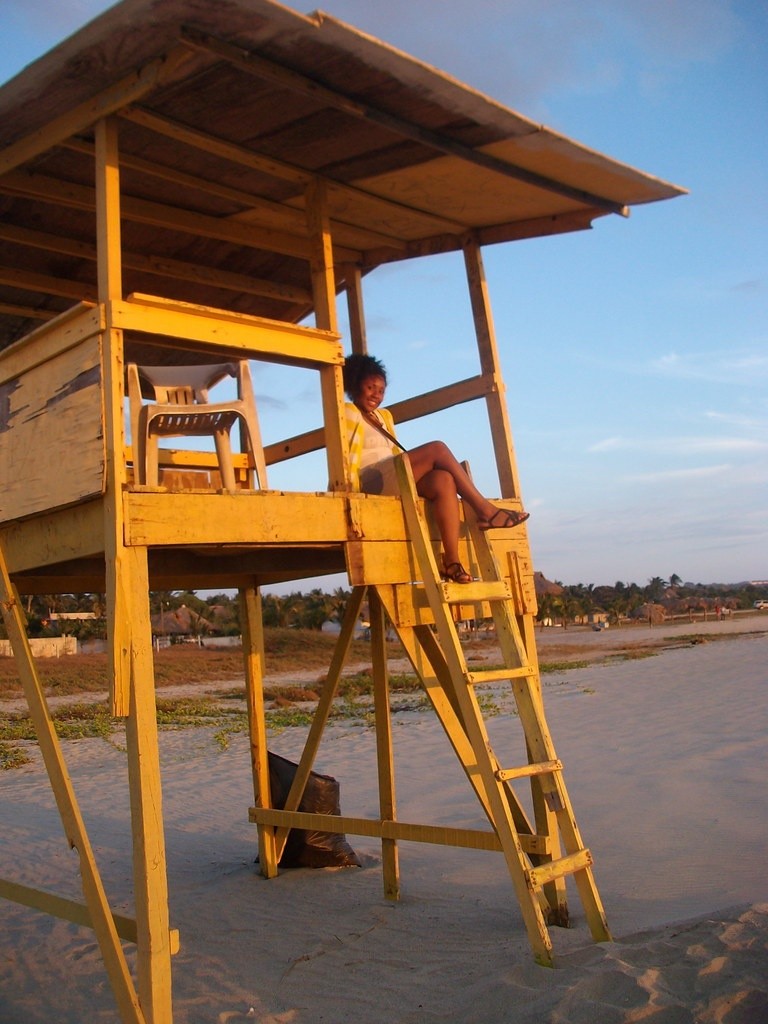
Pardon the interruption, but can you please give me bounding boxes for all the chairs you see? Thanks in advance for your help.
[125,360,267,491]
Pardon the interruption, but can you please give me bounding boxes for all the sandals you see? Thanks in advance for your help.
[439,562,471,584]
[479,508,530,531]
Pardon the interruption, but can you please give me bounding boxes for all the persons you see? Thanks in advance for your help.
[341,352,529,582]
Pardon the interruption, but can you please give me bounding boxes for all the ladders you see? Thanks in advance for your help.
[378,453,613,967]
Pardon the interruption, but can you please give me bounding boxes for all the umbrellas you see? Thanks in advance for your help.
[534,571,563,595]
